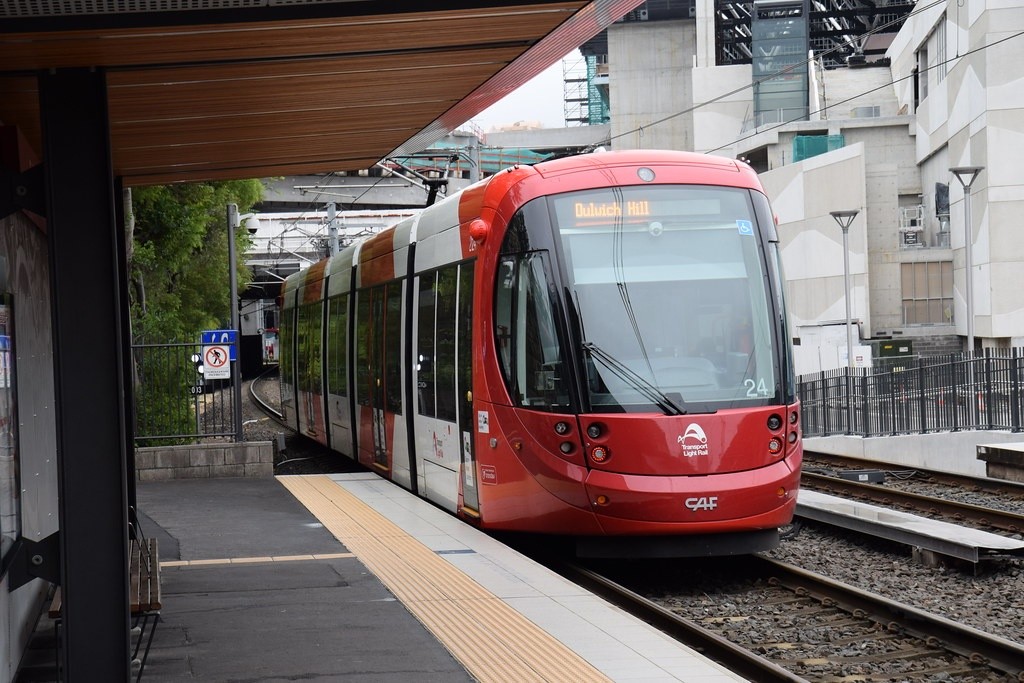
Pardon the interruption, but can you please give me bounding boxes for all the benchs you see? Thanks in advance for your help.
[46,505,163,683]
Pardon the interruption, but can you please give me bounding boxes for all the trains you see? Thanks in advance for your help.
[277,148,803,559]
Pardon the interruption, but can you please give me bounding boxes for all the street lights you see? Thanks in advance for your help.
[829,209,861,435]
[227,203,261,441]
[949,166,984,429]
